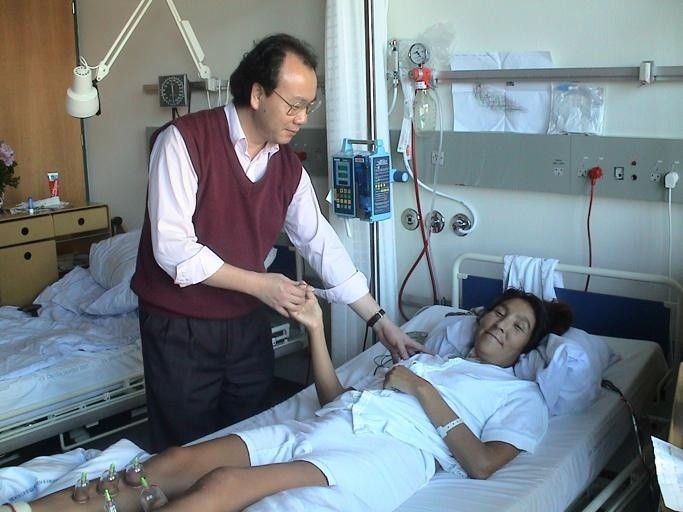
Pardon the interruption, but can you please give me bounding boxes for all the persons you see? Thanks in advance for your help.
[0,286,572,510]
[129,33,438,454]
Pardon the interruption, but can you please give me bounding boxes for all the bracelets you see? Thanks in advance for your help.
[435,417,464,440]
[365,308,385,328]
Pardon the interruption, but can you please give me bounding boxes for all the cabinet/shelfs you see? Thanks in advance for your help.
[0,197,111,308]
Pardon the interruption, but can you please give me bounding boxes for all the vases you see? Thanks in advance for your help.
[0,191,11,212]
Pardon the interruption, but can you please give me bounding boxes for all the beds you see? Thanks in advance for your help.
[0,235,310,464]
[0,252,683,512]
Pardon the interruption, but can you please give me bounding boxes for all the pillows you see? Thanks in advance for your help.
[421,314,622,416]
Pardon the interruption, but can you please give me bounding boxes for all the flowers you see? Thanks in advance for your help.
[0,141,21,194]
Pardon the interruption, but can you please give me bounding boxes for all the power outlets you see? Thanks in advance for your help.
[553,167,679,184]
[431,151,444,166]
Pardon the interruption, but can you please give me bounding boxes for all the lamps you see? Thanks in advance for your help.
[64,0,222,119]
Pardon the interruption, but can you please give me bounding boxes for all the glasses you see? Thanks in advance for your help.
[271,88,322,116]
